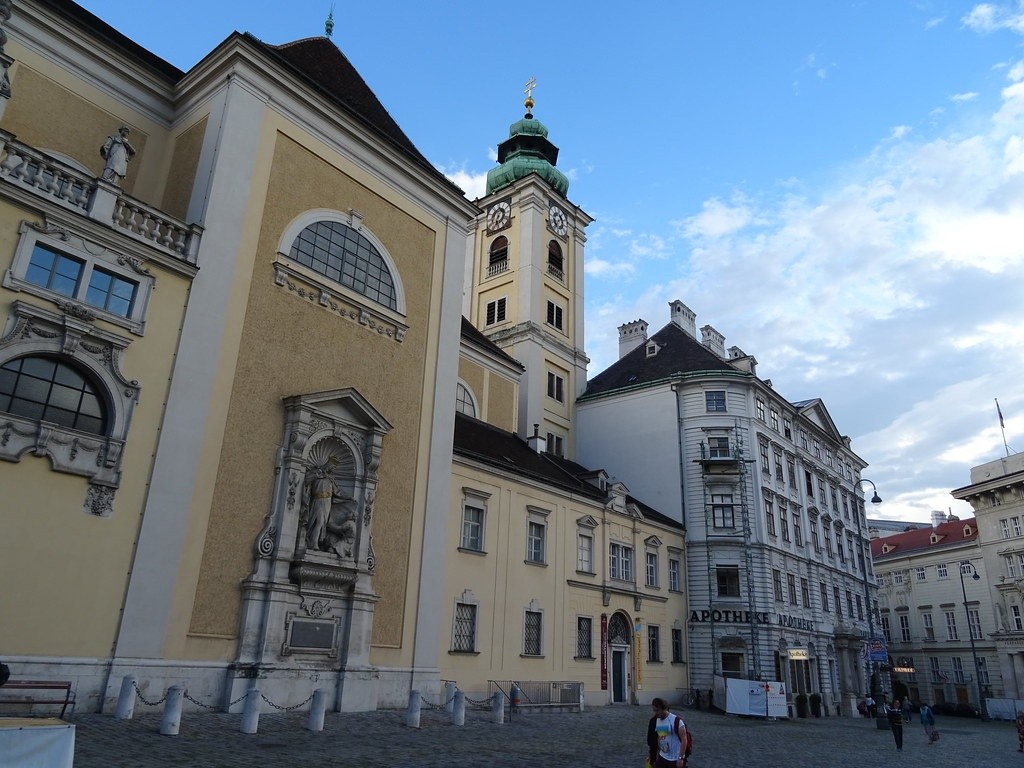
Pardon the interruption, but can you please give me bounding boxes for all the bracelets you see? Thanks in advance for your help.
[678,757,683,760]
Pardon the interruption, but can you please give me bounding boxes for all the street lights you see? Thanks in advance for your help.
[854,479,892,730]
[960,562,992,721]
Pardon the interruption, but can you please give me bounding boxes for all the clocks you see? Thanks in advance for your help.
[486,197,512,237]
[546,200,568,244]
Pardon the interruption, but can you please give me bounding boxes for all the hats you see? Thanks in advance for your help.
[904,696,908,701]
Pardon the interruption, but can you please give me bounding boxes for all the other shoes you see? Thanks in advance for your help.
[910,721,912,724]
[928,738,931,741]
[928,742,932,745]
[1018,749,1023,753]
[897,749,903,752]
[905,721,908,722]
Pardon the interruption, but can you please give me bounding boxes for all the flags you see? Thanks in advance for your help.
[997,407,1004,427]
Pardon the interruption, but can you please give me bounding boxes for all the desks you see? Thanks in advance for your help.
[0,717,76,768]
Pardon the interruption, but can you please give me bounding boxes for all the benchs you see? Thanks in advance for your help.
[0,680,76,722]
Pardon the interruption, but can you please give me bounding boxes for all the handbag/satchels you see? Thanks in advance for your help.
[930,727,940,741]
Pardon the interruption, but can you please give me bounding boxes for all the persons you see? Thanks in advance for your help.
[920,702,935,744]
[647,698,687,768]
[887,699,903,752]
[1015,710,1024,752]
[101,126,136,185]
[306,455,356,551]
[796,690,807,718]
[902,696,912,724]
[809,690,821,718]
[864,694,876,718]
[327,510,357,557]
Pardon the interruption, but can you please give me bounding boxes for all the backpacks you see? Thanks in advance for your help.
[674,716,692,763]
